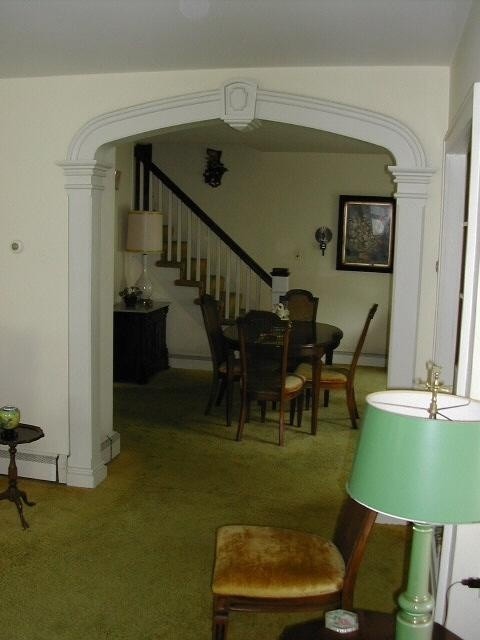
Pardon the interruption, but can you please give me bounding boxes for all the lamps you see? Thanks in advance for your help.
[125,211,163,305]
[203,147,229,188]
[344,369,480,640]
[315,224,332,257]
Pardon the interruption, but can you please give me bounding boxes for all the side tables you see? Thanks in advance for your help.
[276,607,462,640]
[0,423,45,529]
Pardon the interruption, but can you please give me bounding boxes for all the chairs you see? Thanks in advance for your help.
[276,288,319,322]
[194,294,242,426]
[288,303,379,429]
[209,488,378,640]
[232,317,305,446]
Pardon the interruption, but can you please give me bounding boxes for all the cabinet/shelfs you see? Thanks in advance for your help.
[113,299,170,384]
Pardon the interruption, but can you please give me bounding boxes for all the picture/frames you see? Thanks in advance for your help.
[336,194,395,273]
